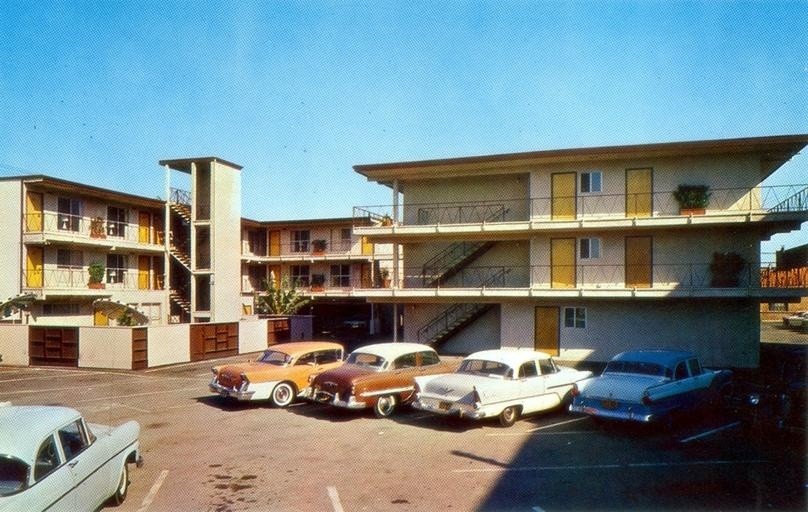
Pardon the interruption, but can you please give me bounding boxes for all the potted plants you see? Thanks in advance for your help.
[710,251,743,289]
[310,238,327,258]
[380,268,392,288]
[89,217,106,240]
[308,273,327,293]
[379,213,394,227]
[86,256,107,289]
[674,184,710,216]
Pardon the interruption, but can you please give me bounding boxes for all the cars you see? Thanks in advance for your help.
[737,348,807,438]
[308,340,450,421]
[213,341,342,408]
[781,309,807,330]
[0,402,146,512]
[568,348,738,435]
[411,349,592,427]
